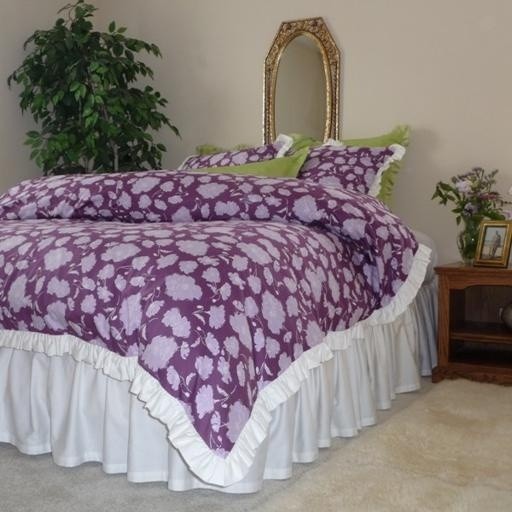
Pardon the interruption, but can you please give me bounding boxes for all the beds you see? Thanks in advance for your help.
[0,122,442,496]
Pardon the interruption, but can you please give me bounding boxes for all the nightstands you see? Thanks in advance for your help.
[419,256,512,388]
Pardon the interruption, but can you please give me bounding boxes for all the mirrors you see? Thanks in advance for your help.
[263,15,341,157]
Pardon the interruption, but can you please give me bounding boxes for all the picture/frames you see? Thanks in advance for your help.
[472,219,512,269]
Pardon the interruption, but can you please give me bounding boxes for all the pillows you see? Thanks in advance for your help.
[173,126,411,225]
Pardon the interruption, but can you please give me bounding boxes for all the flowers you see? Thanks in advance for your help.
[433,164,509,227]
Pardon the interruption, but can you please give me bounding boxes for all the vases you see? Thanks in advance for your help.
[453,215,488,266]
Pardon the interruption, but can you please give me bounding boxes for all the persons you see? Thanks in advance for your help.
[487,229,503,258]
